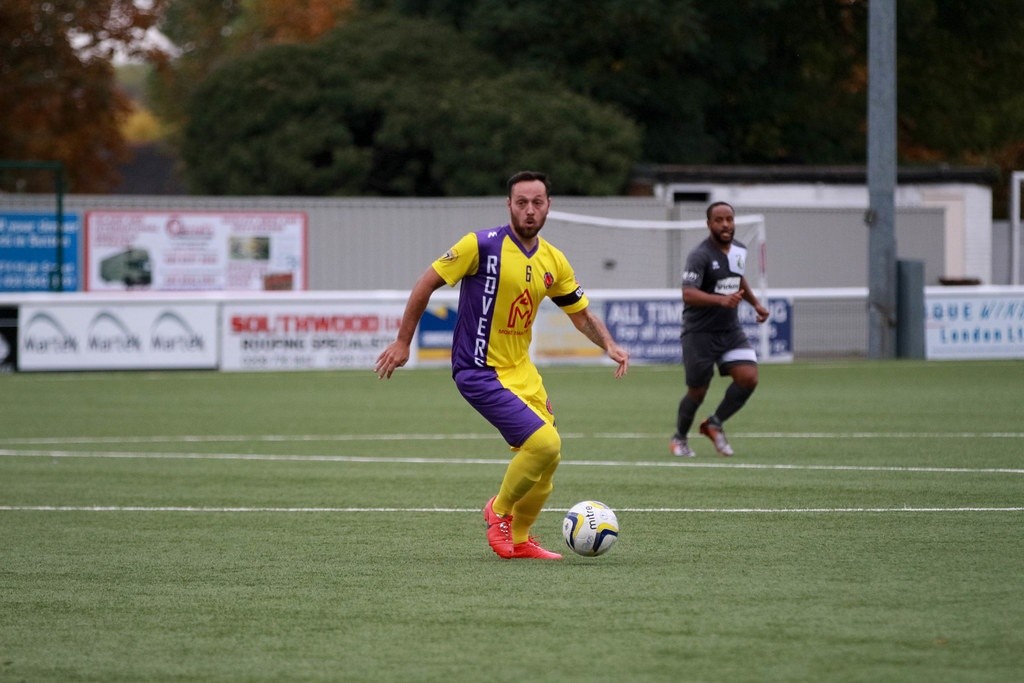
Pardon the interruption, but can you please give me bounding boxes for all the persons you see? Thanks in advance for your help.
[668,202,771,458]
[374,170,629,560]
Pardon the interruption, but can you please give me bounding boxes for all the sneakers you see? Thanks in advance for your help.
[670,434,696,457]
[512,534,563,561]
[483,495,514,560]
[699,414,734,457]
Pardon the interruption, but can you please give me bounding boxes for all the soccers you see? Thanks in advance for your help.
[562,500,619,557]
